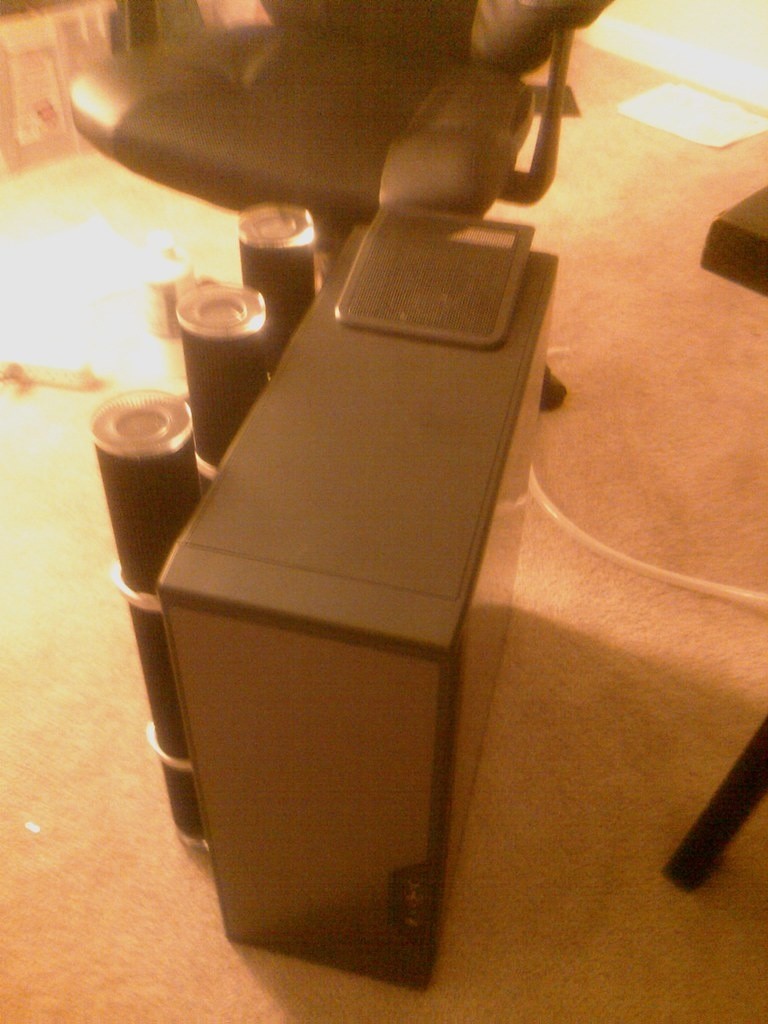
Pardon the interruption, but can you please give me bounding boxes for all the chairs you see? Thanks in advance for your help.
[68,0,613,413]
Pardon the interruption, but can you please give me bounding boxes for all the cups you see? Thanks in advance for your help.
[144,245,219,339]
